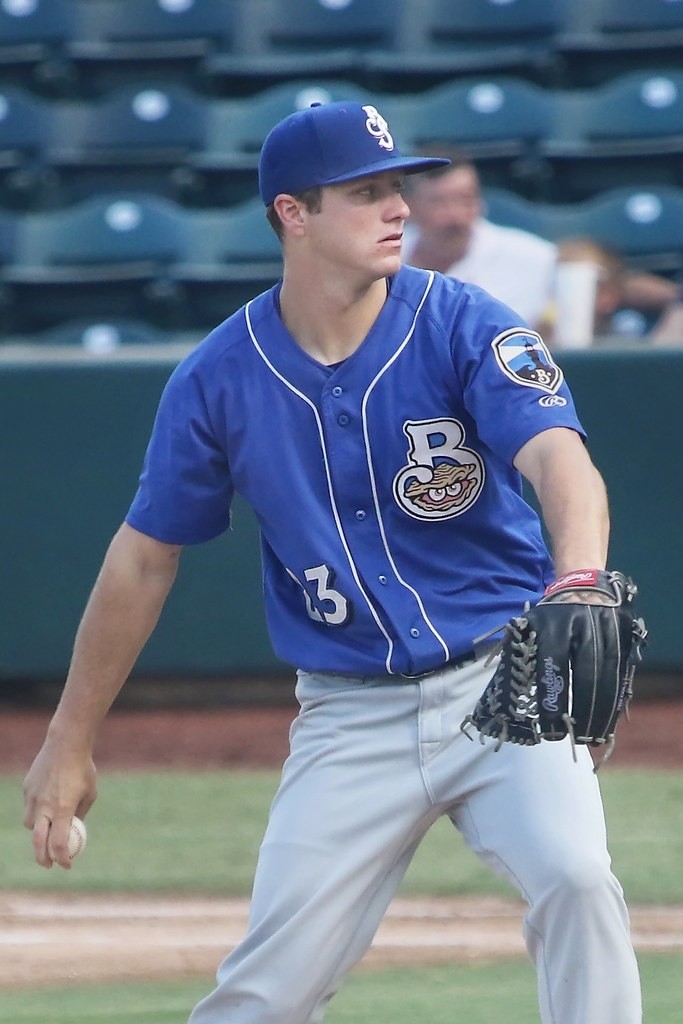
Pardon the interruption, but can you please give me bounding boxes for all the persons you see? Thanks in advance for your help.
[22,102,642,1024]
[398,147,557,345]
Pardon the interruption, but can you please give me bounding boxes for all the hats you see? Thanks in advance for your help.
[257,98,451,208]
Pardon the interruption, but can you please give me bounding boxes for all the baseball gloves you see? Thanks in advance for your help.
[469,567,648,746]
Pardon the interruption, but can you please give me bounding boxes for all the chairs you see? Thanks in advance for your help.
[0,2,683,350]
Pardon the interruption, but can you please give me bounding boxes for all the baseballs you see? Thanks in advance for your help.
[49,815,85,862]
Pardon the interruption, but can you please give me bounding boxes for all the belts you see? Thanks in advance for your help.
[398,650,475,679]
